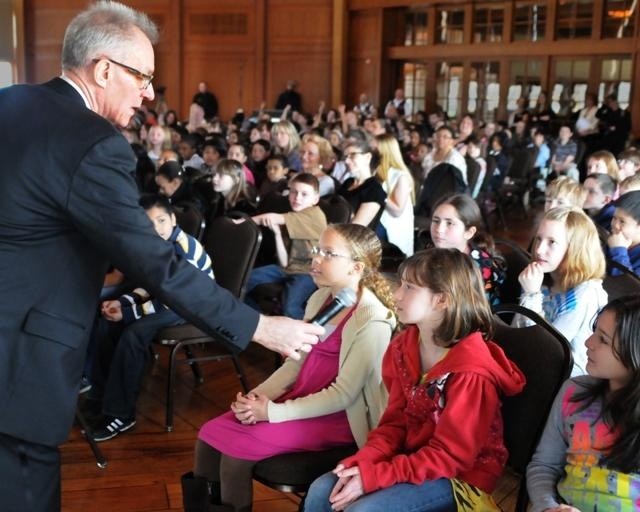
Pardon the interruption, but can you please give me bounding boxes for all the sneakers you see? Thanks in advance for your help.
[91,415,137,442]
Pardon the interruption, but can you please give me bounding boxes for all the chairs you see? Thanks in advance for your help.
[73,145,640,512]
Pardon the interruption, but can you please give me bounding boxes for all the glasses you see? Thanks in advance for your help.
[91,55,156,90]
[310,244,351,262]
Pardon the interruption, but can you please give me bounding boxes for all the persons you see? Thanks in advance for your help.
[77,79,639,512]
[0,1,326,512]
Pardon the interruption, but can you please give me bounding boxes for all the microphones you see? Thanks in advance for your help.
[304,288,356,329]
